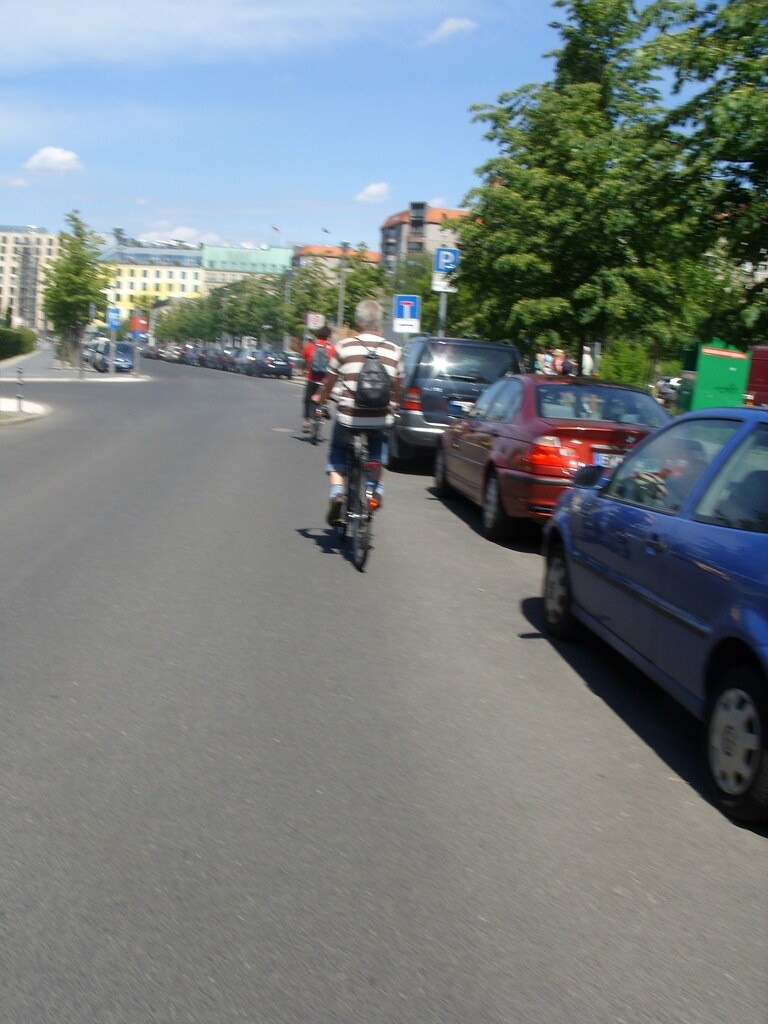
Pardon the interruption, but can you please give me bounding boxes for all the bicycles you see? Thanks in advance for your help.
[301,380,330,445]
[312,394,400,571]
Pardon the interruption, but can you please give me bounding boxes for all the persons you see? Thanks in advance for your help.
[326,299,406,527]
[581,345,594,376]
[534,346,578,375]
[302,325,336,433]
[547,386,604,420]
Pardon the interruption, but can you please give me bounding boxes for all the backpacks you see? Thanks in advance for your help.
[338,338,392,409]
[306,341,329,376]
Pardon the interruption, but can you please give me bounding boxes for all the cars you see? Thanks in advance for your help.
[283,351,305,364]
[82,337,135,371]
[140,345,260,373]
[436,373,705,535]
[655,376,682,400]
[252,349,292,379]
[541,406,767,809]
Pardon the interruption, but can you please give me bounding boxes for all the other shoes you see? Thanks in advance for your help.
[327,493,344,527]
[320,406,331,421]
[302,423,312,433]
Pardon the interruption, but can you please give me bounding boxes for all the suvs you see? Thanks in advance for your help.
[381,331,526,470]
